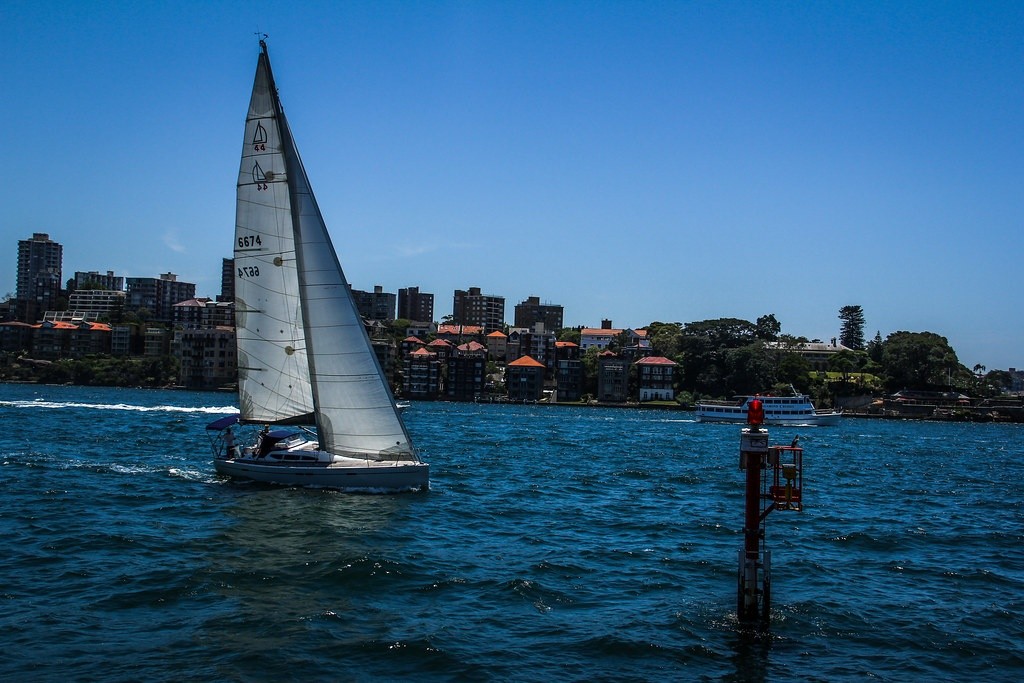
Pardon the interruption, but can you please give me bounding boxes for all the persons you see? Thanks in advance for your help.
[258,424,270,441]
[223,427,236,458]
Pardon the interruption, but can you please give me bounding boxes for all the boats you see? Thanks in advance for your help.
[690,385,844,426]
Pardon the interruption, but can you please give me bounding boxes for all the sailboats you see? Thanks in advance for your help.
[205,32,430,489]
